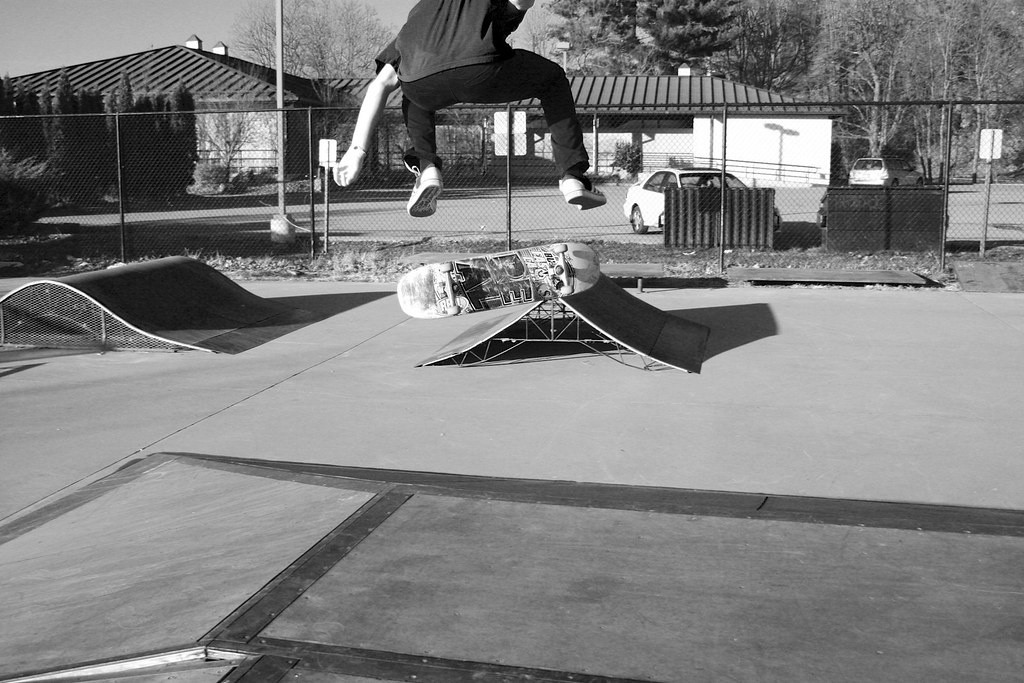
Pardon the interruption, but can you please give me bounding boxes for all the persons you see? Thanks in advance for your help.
[333,0,607,217]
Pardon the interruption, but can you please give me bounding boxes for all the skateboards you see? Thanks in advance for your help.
[395,241,603,319]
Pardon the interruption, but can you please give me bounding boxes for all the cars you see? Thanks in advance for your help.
[624,167,781,235]
[849,157,929,189]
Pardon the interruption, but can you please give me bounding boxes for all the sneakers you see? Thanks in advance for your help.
[561,175,607,210]
[404,160,443,217]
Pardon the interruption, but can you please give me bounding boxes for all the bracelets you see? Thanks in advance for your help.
[348,145,368,157]
